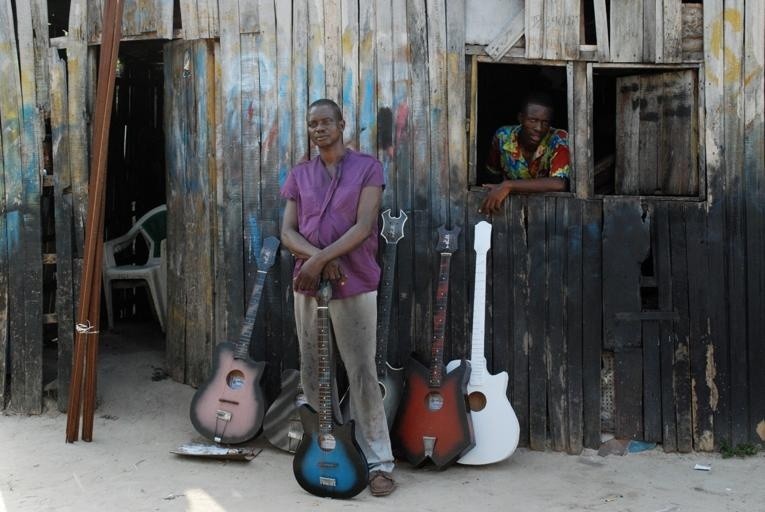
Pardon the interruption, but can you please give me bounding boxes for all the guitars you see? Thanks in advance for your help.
[262,367,303,454]
[189,236,282,446]
[338,209,408,437]
[389,224,476,471]
[293,279,370,500]
[447,220,520,466]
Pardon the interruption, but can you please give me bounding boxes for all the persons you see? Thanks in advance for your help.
[476,103,570,218]
[278,100,399,496]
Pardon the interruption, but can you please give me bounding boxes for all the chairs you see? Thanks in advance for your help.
[103,204,168,334]
